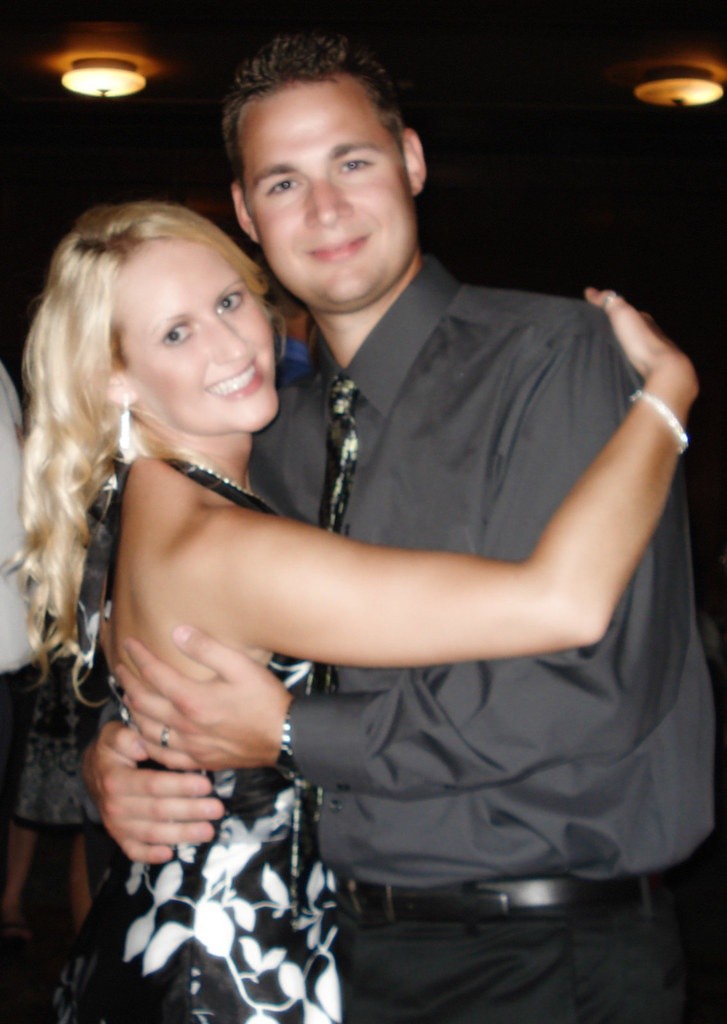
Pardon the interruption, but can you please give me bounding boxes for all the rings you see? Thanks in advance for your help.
[601,293,619,310]
[161,726,168,748]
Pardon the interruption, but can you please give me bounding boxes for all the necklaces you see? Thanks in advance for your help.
[186,461,264,504]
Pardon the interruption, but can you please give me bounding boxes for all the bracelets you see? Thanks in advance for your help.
[629,391,689,452]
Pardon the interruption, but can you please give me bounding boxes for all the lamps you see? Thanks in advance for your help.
[601,25,727,106]
[33,13,184,99]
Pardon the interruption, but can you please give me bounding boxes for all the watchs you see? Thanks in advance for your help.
[277,702,300,781]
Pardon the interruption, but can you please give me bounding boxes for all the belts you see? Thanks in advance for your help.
[340,868,688,926]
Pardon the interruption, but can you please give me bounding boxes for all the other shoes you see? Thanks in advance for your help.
[0,921,32,949]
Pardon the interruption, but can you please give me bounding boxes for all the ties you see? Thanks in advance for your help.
[286,376,360,977]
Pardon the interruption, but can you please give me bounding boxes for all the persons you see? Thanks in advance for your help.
[0,200,700,1024]
[1,262,315,945]
[82,31,716,1024]
